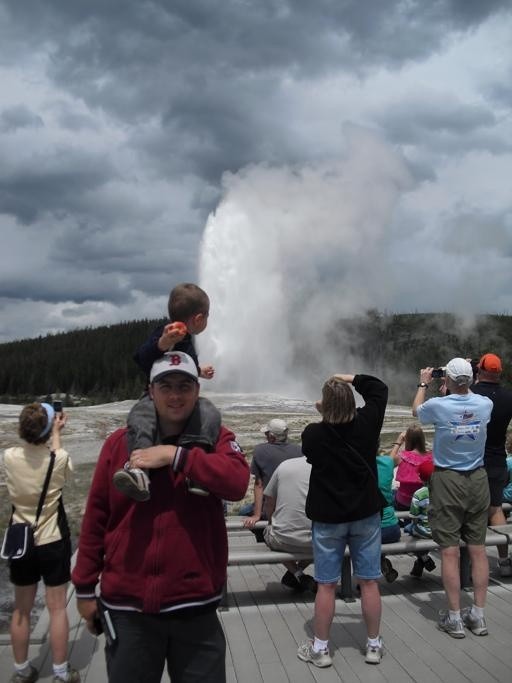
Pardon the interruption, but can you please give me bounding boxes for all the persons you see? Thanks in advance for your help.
[245,415,511,595]
[438,351,511,577]
[410,355,497,639]
[109,282,222,502]
[70,349,251,682]
[295,371,390,668]
[0,401,84,682]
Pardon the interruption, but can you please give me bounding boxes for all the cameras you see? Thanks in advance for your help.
[471,360,480,373]
[429,367,447,378]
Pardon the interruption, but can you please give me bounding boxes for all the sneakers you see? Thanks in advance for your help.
[365,637,385,663]
[461,606,488,635]
[380,556,398,583]
[186,477,209,495]
[496,559,511,575]
[11,666,40,682]
[297,638,332,668]
[436,609,465,638]
[112,467,150,501]
[410,560,423,577]
[424,555,436,571]
[298,573,313,589]
[281,568,306,589]
[54,665,80,682]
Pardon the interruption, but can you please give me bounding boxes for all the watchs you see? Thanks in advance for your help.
[415,382,428,388]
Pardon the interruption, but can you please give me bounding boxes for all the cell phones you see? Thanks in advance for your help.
[98,608,116,647]
[52,399,63,419]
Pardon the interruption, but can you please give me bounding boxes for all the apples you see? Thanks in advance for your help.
[167,322,186,337]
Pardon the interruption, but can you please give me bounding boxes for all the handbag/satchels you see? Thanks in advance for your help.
[2,523,37,566]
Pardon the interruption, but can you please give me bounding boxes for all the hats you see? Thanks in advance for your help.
[415,460,434,480]
[260,418,289,435]
[476,352,501,372]
[150,351,199,383]
[443,357,473,385]
